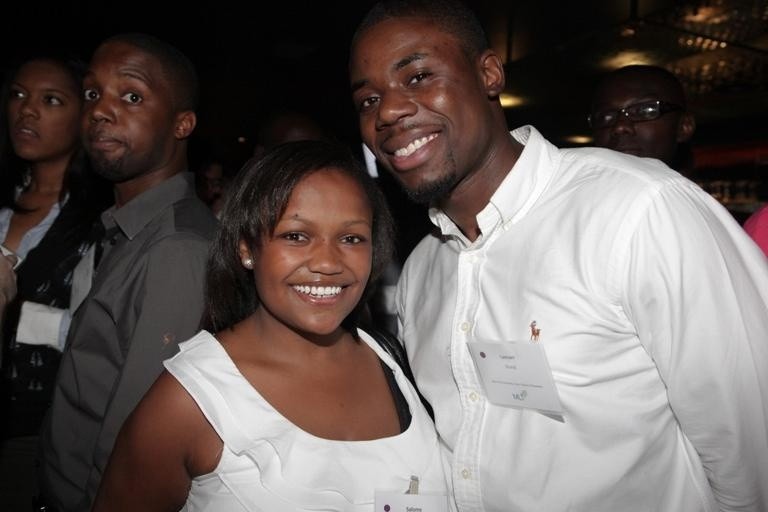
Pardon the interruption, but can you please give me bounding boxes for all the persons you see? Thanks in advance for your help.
[92,148,450,511]
[0,3,215,512]
[351,4,767,512]
[193,99,392,334]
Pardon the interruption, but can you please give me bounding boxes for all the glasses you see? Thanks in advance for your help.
[585,100,682,131]
